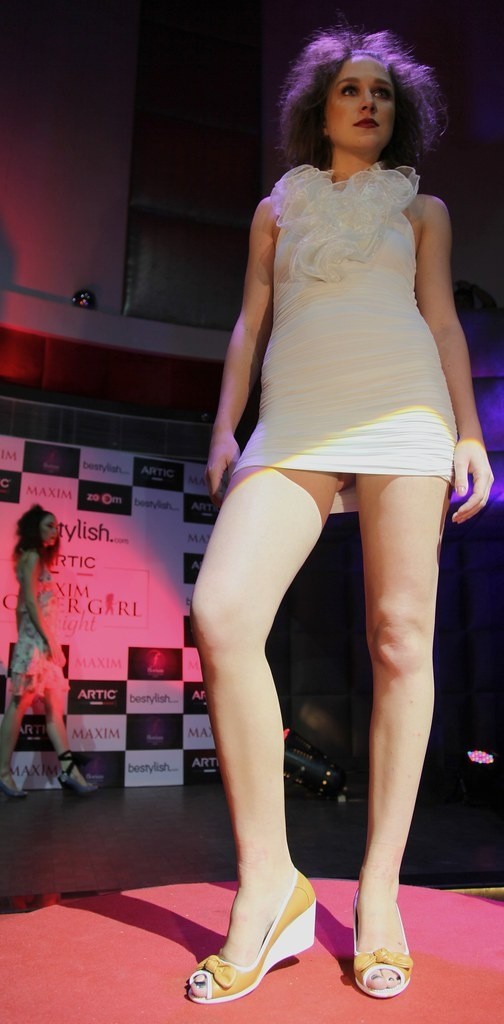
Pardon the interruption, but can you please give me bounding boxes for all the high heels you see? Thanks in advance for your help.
[58,749,101,794]
[0,779,28,797]
[351,889,414,998]
[187,867,316,1003]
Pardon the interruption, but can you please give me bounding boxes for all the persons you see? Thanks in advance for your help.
[184,31,495,1005]
[0,504,101,797]
